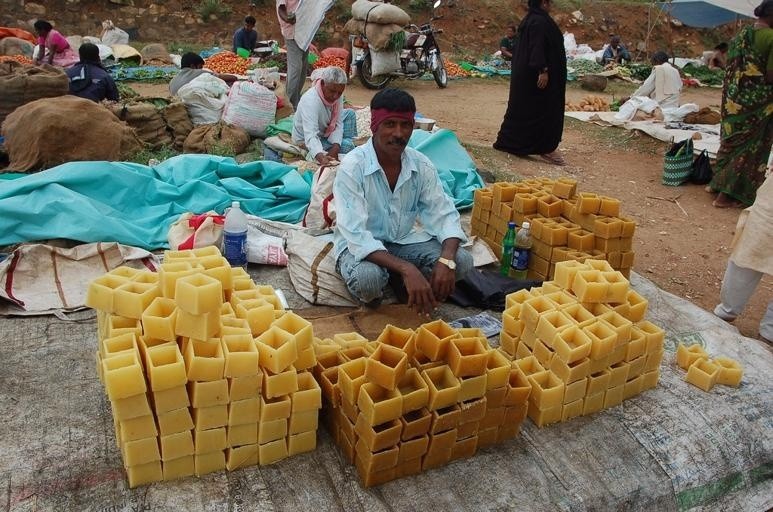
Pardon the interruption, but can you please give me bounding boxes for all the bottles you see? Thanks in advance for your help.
[221,200,250,273]
[510,221,531,279]
[499,221,515,277]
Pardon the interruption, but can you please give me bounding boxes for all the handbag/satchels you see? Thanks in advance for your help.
[283,223,361,309]
[303,163,335,235]
[0,239,164,323]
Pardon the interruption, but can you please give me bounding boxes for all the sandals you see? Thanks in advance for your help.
[541,152,566,166]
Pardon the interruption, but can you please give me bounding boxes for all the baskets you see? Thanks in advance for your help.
[662,135,694,187]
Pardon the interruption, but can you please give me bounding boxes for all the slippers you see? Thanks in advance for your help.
[713,200,737,208]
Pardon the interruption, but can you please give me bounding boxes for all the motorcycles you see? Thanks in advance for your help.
[348,1,448,90]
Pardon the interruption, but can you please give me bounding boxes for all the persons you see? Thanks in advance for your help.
[708,42,730,76]
[619,52,684,112]
[33,20,79,68]
[292,64,358,167]
[602,33,633,67]
[713,141,773,346]
[232,15,259,57]
[494,0,567,164]
[704,1,773,209]
[65,43,120,103]
[500,24,518,61]
[333,87,473,318]
[275,1,334,110]
[168,53,238,95]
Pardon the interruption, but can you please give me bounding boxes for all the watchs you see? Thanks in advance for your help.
[439,257,459,271]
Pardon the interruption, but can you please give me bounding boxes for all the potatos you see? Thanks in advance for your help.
[564,94,609,113]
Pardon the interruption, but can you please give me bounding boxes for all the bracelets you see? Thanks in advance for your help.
[35,55,40,60]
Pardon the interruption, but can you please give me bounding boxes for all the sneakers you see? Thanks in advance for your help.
[388,272,408,304]
